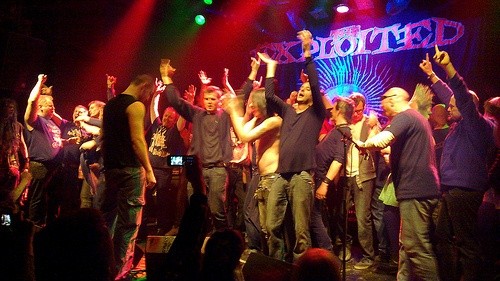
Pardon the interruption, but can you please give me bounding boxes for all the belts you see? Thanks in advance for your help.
[204,163,228,168]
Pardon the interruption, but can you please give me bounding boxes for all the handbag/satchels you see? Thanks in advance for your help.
[30,161,47,180]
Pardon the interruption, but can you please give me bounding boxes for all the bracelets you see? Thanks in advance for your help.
[428,72,436,80]
[24,157,29,163]
[323,177,332,185]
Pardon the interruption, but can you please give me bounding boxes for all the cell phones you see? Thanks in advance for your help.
[167,155,194,166]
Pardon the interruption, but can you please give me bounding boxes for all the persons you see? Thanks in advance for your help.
[35,208,116,281]
[292,247,342,281]
[419,45,500,281]
[315,93,400,271]
[100,74,157,281]
[144,59,282,252]
[0,73,117,228]
[257,31,326,264]
[156,155,244,281]
[356,87,441,281]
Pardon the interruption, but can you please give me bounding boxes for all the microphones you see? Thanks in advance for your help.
[356,143,369,158]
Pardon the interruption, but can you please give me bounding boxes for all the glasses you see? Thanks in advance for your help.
[354,108,363,114]
[380,94,396,101]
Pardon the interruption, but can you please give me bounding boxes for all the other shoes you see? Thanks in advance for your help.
[339,245,352,261]
[353,254,380,269]
[165,225,179,236]
[239,248,257,263]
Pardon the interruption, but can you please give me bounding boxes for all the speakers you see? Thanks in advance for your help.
[242,252,293,281]
[145,235,210,281]
